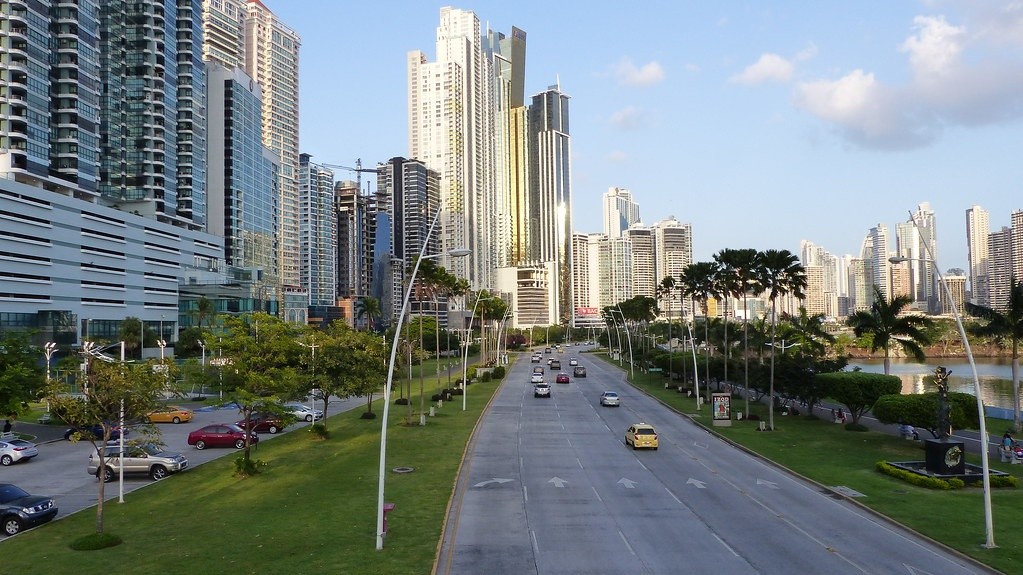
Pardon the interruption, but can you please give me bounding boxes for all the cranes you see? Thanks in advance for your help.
[322,158,377,195]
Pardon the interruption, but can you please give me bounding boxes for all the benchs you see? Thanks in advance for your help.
[899,430,921,443]
[999,448,1023,465]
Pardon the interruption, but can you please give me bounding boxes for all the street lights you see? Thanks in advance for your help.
[765,339,802,397]
[92,342,124,503]
[530,316,550,348]
[497,301,519,368]
[888,209,999,549]
[45,342,59,412]
[672,336,697,388]
[157,340,166,390]
[78,341,104,415]
[403,340,417,380]
[463,280,494,410]
[589,307,622,367]
[293,332,319,425]
[646,334,663,348]
[661,280,700,411]
[376,202,471,550]
[609,304,633,380]
[197,339,208,393]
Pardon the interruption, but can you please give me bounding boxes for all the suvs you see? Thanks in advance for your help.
[87,439,189,482]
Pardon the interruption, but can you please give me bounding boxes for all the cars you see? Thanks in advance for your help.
[188,424,259,449]
[0,439,38,466]
[64,420,129,441]
[0,484,58,536]
[534,383,551,398]
[600,391,619,407]
[278,404,323,422]
[625,423,658,451]
[574,366,586,377]
[140,406,193,424]
[556,373,570,384]
[235,412,284,434]
[531,341,594,383]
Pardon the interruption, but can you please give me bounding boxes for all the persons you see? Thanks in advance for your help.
[831,407,846,423]
[799,399,806,408]
[4,420,11,435]
[1003,431,1021,452]
[898,424,921,440]
